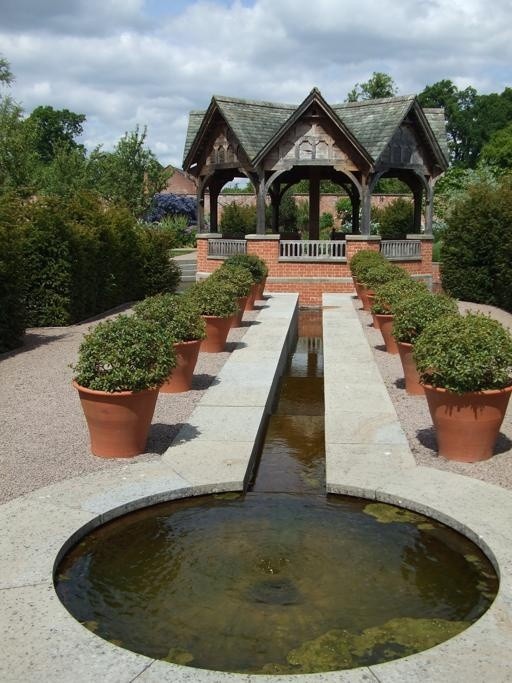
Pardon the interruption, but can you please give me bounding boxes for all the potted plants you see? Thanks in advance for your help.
[73,248,267,462]
[350,247,511,464]
